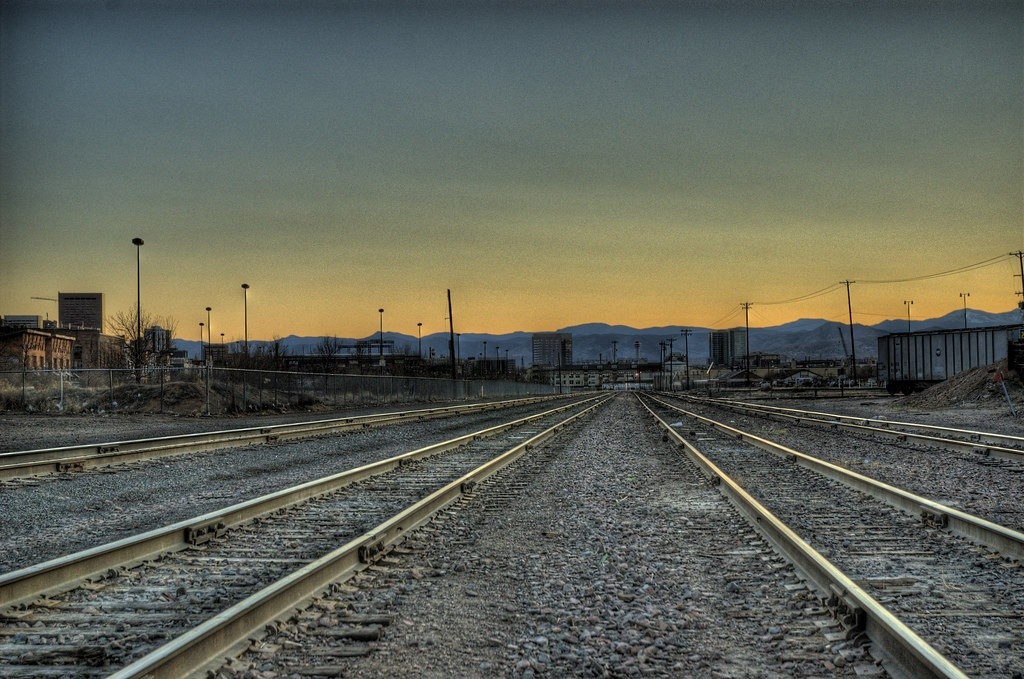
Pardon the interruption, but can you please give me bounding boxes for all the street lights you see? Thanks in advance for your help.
[457,334,461,365]
[379,309,384,356]
[496,347,500,368]
[960,293,971,329]
[236,341,239,353]
[505,350,509,365]
[418,323,422,357]
[241,284,250,358]
[904,300,914,332]
[198,322,204,380]
[206,308,212,361]
[221,333,224,359]
[131,238,144,383]
[483,341,487,359]
